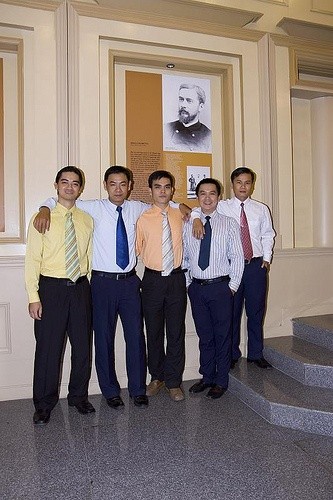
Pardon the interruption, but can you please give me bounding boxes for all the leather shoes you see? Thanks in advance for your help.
[129,392,148,409]
[164,383,185,402]
[230,358,238,369]
[145,380,165,397]
[246,356,273,369]
[33,408,51,426]
[106,395,125,409]
[206,384,228,399]
[188,381,215,393]
[68,396,96,415]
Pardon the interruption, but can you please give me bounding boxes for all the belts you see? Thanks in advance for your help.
[192,275,229,286]
[243,257,260,264]
[93,270,136,281]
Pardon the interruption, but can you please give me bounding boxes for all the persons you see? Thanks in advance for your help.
[33,166,192,408]
[190,167,276,371]
[136,171,187,402]
[24,166,94,425]
[181,178,244,399]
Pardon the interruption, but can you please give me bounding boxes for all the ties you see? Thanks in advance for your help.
[115,206,129,271]
[65,212,81,283]
[239,203,254,260]
[198,216,212,272]
[161,212,174,277]
